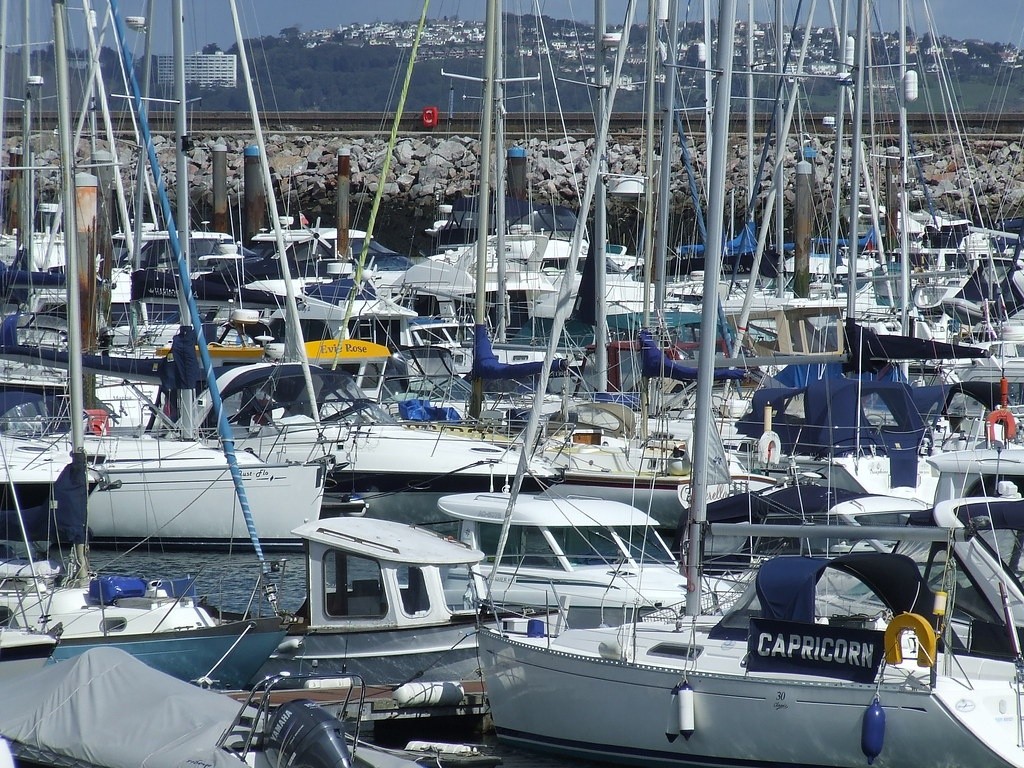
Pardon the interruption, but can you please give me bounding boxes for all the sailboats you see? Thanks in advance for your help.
[0,0,1024,768]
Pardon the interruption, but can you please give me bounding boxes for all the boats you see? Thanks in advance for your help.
[49,517,572,727]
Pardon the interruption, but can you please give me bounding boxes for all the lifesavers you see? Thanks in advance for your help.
[759,431,782,465]
[884,613,937,667]
[984,410,1016,440]
